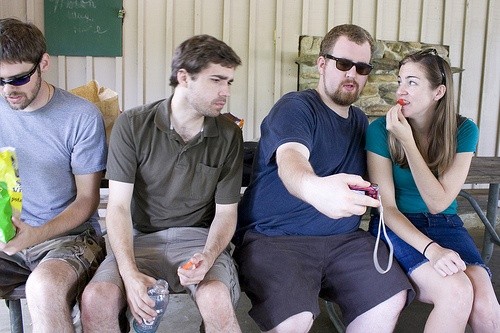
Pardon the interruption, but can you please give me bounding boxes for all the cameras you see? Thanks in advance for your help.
[349,182,379,200]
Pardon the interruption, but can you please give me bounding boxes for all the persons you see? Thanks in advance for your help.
[230,24,416,333]
[79,34,243,333]
[0,19,108,333]
[365,48,500,333]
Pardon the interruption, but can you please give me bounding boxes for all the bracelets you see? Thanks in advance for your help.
[421,240,436,257]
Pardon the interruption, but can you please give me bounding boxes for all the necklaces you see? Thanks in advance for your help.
[42,78,53,103]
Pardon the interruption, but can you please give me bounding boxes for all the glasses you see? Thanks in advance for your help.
[398,48,446,86]
[0,54,44,86]
[323,53,374,76]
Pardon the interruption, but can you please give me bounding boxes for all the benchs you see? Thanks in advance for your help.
[2,141,500,333]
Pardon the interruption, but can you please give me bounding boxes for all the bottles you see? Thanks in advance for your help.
[131,280,170,333]
